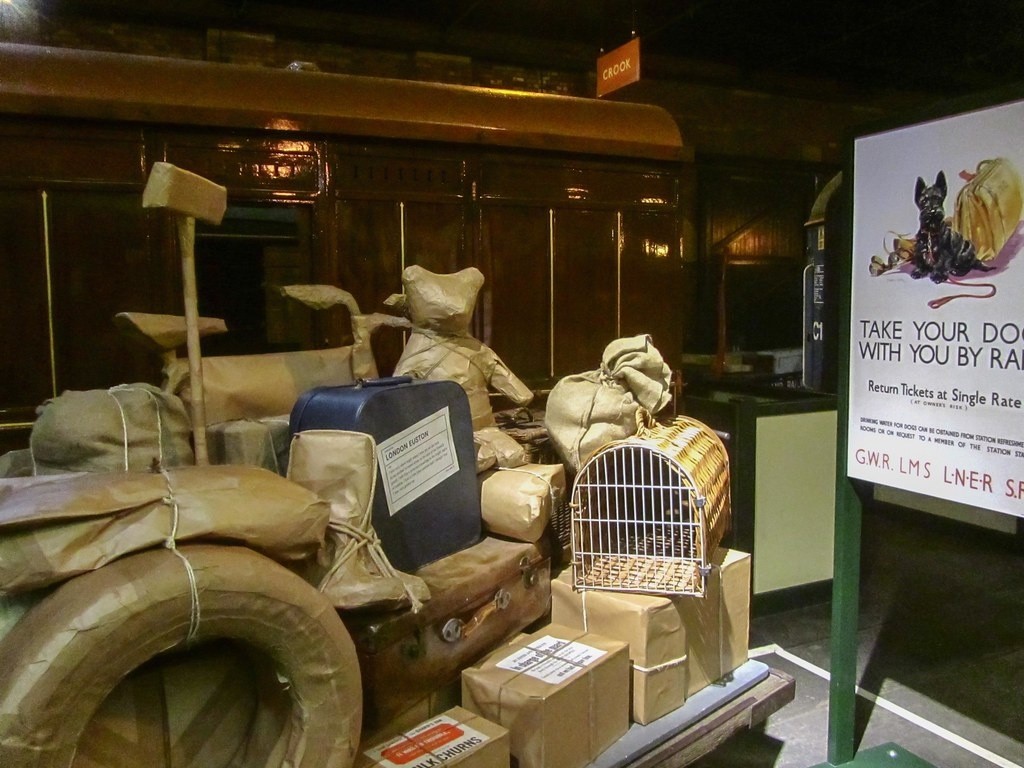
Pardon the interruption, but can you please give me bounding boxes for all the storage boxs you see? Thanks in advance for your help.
[458,624,630,768]
[351,702,510,768]
[550,545,753,726]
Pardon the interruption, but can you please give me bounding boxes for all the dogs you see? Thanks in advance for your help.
[912,171,998,284]
